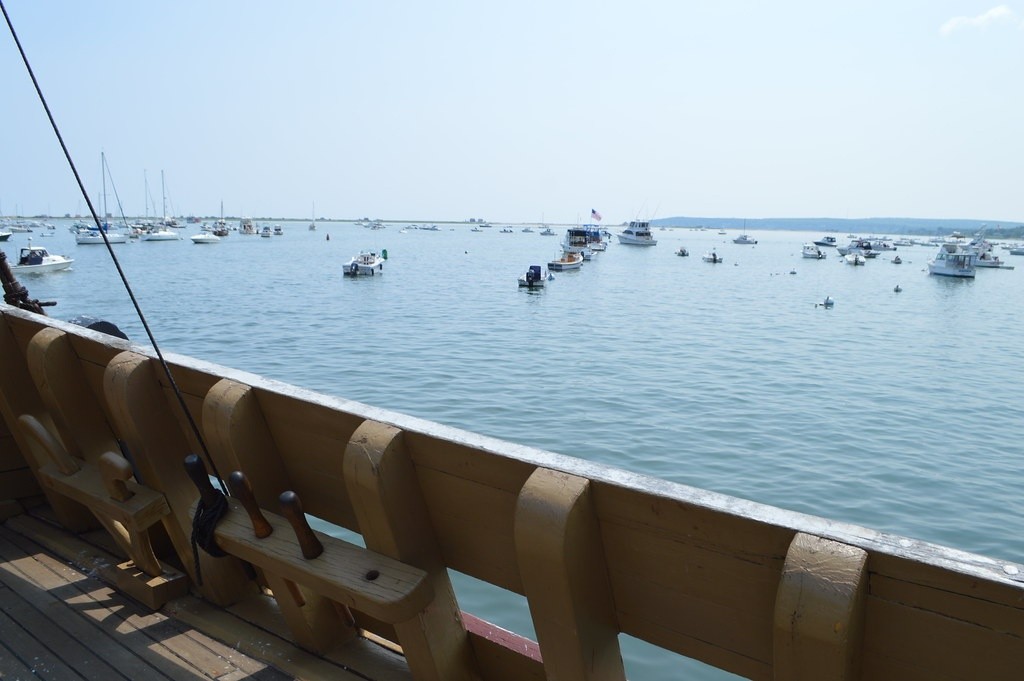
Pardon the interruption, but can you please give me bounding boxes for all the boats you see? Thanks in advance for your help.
[500,229,513,233]
[677,247,689,256]
[8,237,75,275]
[355,220,385,229]
[518,266,550,288]
[404,225,439,230]
[538,225,549,228]
[200,201,283,237]
[617,220,658,245]
[702,251,723,263]
[540,229,557,235]
[522,228,533,232]
[309,224,316,230]
[480,223,492,227]
[191,232,220,243]
[548,224,611,270]
[732,219,758,244]
[1,217,56,240]
[342,251,385,276]
[800,224,1024,305]
[471,228,482,232]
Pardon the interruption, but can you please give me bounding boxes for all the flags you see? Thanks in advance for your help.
[591,209,602,222]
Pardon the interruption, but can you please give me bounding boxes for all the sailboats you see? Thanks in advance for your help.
[68,152,187,244]
